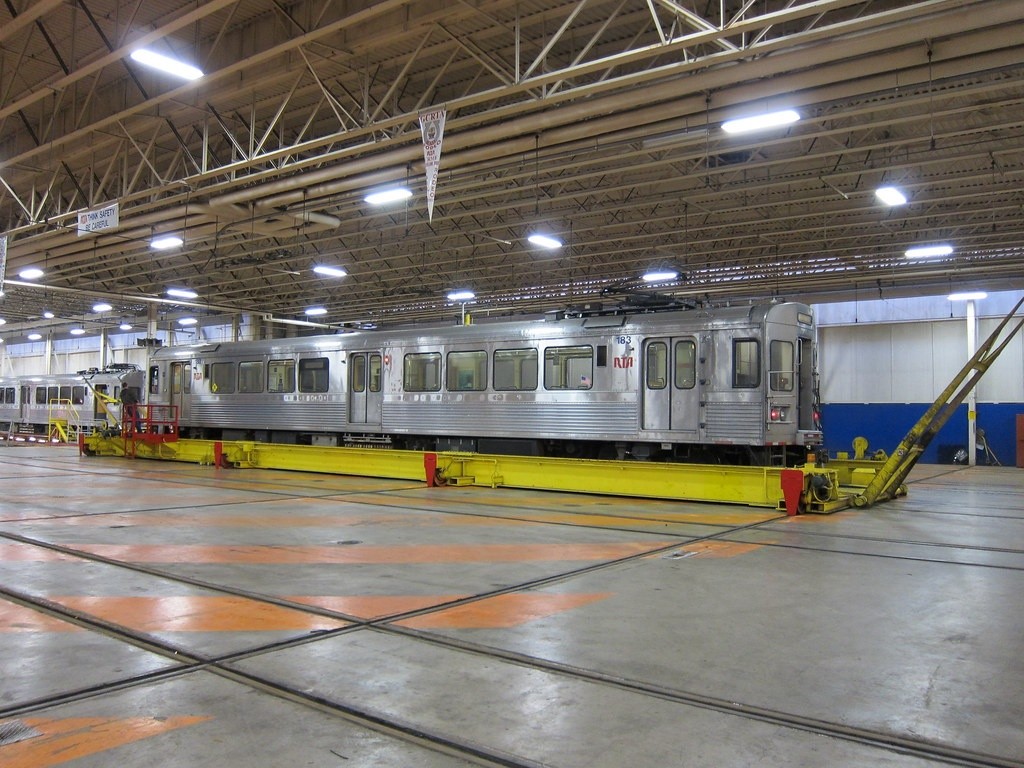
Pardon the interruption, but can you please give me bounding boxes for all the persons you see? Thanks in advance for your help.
[120,383,142,433]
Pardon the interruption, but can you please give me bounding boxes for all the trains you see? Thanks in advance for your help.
[0,298,829,468]
